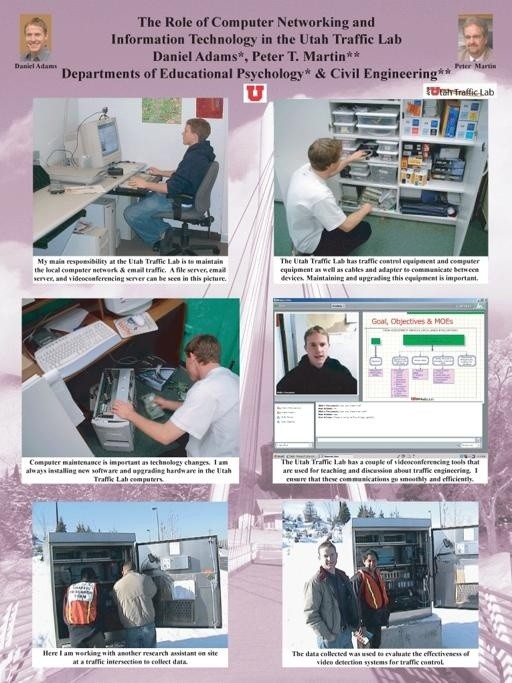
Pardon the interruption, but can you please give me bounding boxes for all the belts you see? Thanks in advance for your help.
[341,623,350,632]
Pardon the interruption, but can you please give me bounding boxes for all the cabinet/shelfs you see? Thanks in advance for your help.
[274,99,487,254]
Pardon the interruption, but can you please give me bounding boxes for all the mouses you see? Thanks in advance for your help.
[132,314,144,326]
[145,169,153,174]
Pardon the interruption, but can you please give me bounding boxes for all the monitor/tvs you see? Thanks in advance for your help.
[64,117,122,168]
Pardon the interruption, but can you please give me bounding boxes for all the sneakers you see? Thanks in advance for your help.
[159,229,173,256]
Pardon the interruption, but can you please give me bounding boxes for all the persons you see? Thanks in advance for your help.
[122,117,215,255]
[459,17,493,62]
[285,137,373,256]
[20,18,52,61]
[303,540,361,648]
[63,568,106,648]
[113,561,157,648]
[276,326,357,393]
[109,333,240,457]
[349,550,391,648]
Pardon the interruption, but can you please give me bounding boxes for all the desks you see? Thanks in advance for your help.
[33,158,147,249]
[22,297,186,389]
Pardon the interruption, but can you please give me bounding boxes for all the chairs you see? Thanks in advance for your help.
[152,160,224,255]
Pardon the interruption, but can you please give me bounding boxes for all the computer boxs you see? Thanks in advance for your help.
[91,368,136,453]
[83,198,117,256]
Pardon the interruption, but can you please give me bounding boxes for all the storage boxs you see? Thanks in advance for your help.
[331,108,398,183]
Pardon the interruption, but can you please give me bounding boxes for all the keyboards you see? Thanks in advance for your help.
[34,320,121,380]
[119,173,156,189]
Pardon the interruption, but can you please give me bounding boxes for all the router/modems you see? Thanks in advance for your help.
[49,184,65,194]
